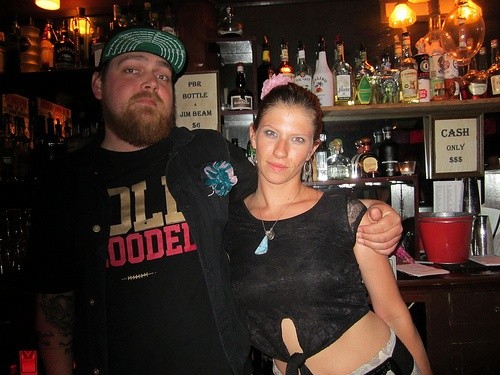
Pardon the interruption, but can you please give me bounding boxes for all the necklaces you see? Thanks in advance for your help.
[255,186,302,254]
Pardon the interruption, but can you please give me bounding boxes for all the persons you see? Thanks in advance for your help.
[37,29,403,375]
[222,74,432,375]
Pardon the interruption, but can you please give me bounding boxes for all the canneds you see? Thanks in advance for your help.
[415,52,461,103]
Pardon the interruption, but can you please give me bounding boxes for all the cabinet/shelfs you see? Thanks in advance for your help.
[220,97,500,375]
[0,66,99,164]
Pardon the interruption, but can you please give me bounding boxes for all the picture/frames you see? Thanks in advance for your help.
[428,111,485,179]
[175,70,222,132]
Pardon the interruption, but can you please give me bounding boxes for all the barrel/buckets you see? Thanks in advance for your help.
[416,212,473,264]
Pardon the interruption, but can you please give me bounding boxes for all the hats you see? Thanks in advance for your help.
[98,27,186,81]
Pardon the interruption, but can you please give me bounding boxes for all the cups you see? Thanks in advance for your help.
[398,161,416,175]
[472,215,495,257]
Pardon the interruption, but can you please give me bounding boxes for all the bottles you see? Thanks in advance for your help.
[0,0,179,276]
[225,0,500,110]
[312,126,394,181]
